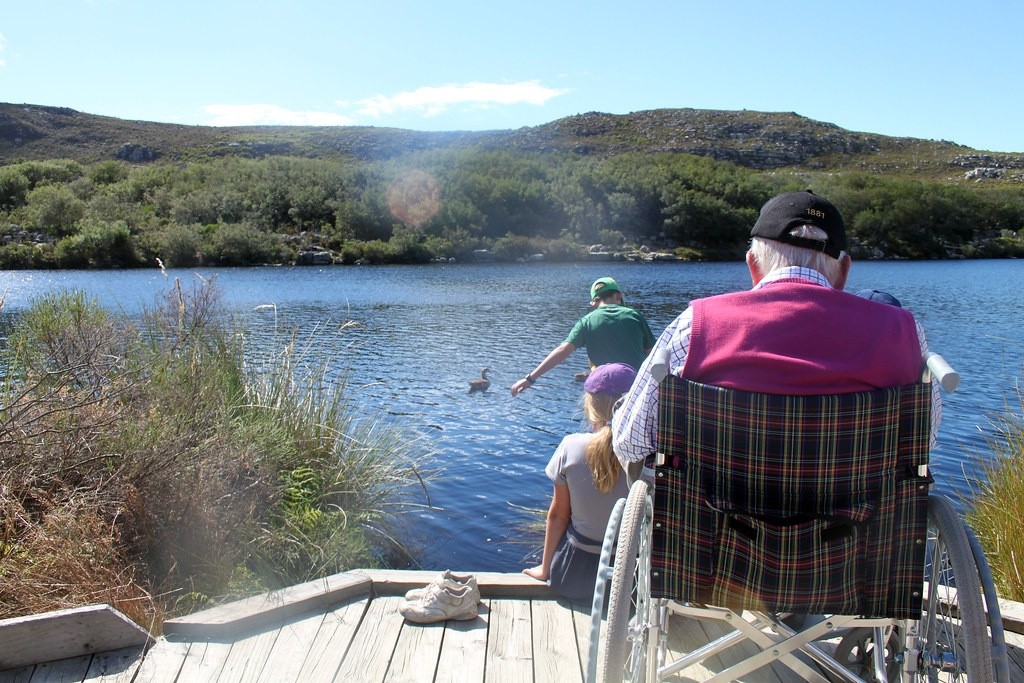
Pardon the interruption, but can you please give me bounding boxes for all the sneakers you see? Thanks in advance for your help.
[398,585,478,623]
[405,570,481,604]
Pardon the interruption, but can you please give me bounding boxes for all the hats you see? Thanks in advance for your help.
[852,290,902,308]
[590,277,621,299]
[584,363,637,396]
[751,190,846,259]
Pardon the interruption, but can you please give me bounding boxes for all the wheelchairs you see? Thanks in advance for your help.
[586,347,1011,683]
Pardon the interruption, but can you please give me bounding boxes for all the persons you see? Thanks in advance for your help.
[510,277,656,397]
[613,191,941,467]
[524,362,640,607]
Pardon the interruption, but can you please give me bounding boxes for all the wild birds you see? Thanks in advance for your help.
[468,367,491,391]
[575,372,589,382]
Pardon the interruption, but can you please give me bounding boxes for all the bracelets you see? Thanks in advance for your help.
[525,375,534,385]
